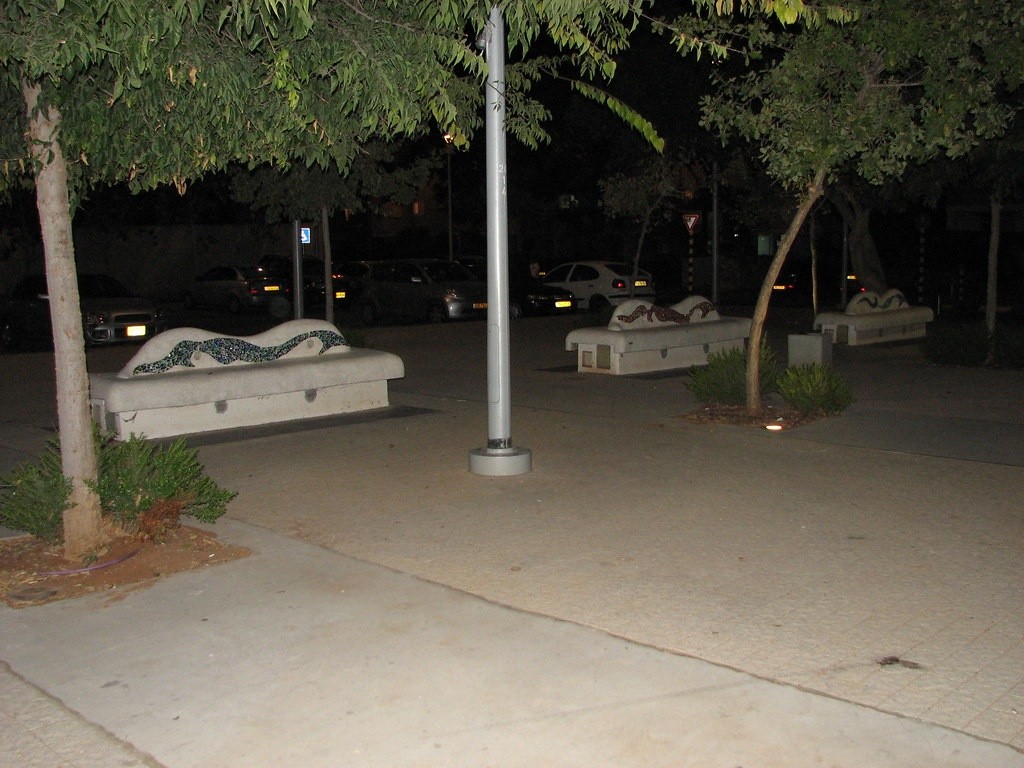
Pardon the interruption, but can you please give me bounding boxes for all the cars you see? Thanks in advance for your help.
[770,259,825,308]
[181,262,280,315]
[285,257,578,328]
[77,274,172,348]
[0,285,52,353]
[540,260,657,315]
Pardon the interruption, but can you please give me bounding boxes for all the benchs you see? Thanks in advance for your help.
[88,320,406,442]
[564,295,756,377]
[812,289,934,356]
